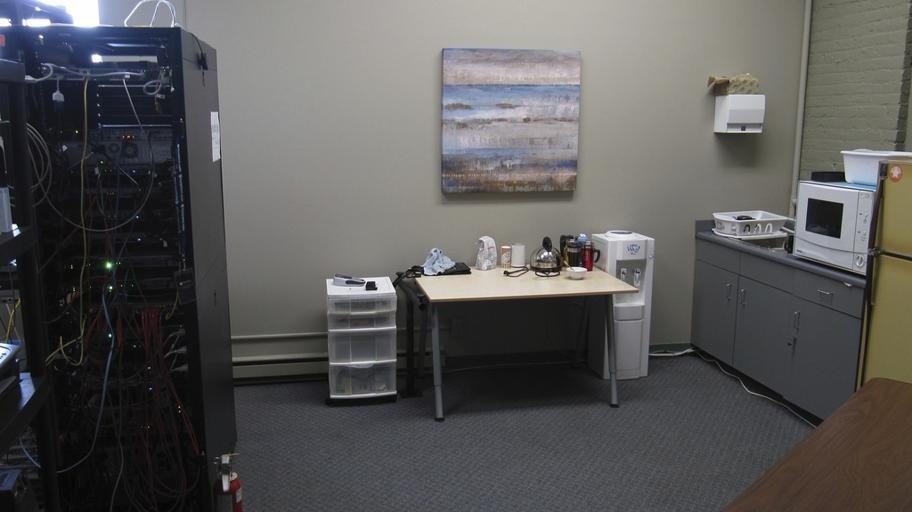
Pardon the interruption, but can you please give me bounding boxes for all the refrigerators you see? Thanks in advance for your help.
[854,160,911,390]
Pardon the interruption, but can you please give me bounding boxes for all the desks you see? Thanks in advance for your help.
[415,264,640,421]
[721,377,912,512]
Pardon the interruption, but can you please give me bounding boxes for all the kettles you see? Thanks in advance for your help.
[531,236,564,272]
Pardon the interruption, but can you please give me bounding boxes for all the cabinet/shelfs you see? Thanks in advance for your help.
[690,220,867,421]
[326,276,397,402]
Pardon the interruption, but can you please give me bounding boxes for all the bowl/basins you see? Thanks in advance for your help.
[566,266,587,280]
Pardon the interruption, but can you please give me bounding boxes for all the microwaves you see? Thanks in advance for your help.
[793,180,878,277]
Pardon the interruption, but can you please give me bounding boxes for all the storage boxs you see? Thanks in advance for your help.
[842,150,911,185]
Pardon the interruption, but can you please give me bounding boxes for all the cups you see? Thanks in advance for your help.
[559,232,601,271]
